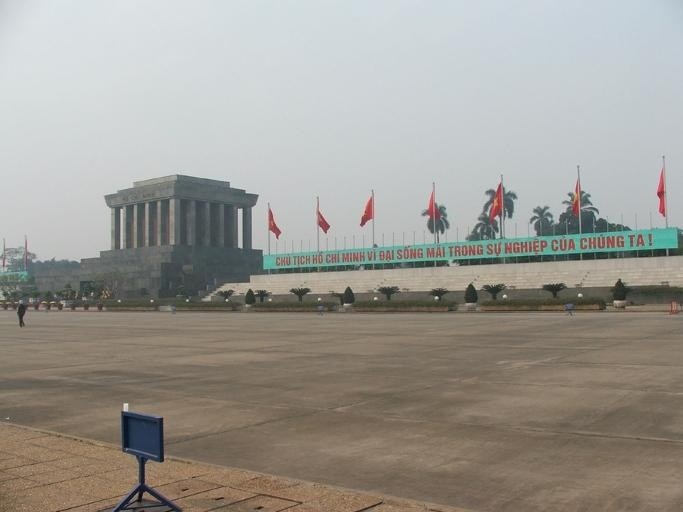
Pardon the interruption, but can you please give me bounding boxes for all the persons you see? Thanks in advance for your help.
[16,299,25,327]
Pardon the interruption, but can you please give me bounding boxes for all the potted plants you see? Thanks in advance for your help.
[242,288,257,311]
[0,278,111,311]
[609,275,629,310]
[339,284,356,312]
[461,282,480,312]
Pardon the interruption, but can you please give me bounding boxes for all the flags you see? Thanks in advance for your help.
[655,170,665,216]
[429,185,441,220]
[267,207,282,240]
[489,184,502,223]
[573,175,581,217]
[360,195,371,226]
[317,204,330,235]
